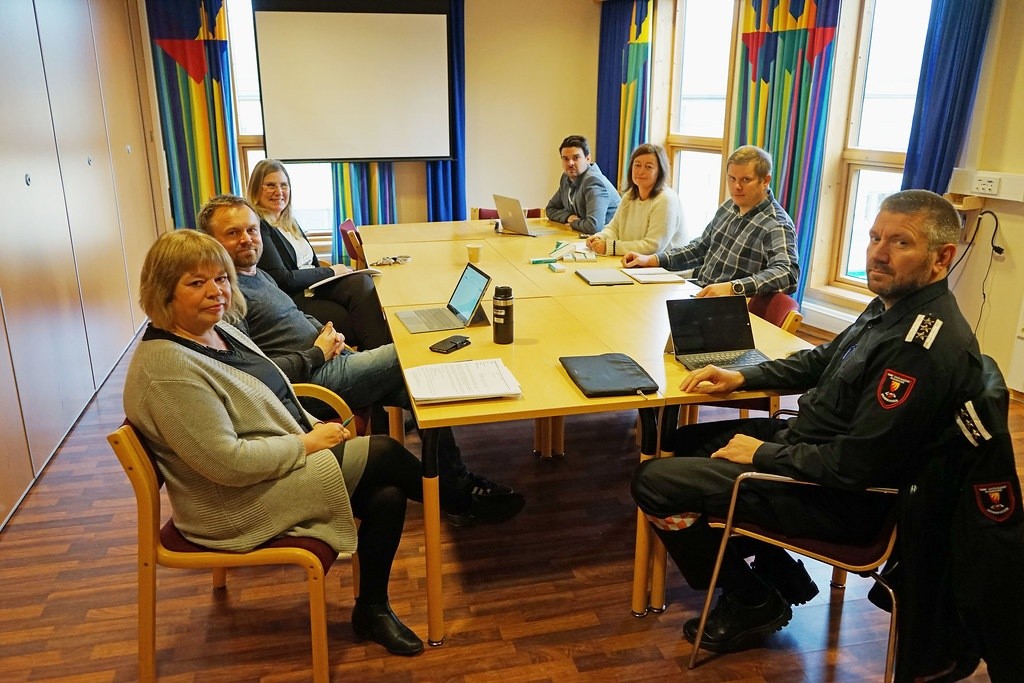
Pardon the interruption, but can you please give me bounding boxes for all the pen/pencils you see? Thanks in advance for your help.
[341,415,354,427]
[690,294,697,297]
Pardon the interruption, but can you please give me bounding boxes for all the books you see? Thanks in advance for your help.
[575,269,633,285]
[621,267,685,284]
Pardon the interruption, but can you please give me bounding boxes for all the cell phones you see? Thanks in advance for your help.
[431,339,468,353]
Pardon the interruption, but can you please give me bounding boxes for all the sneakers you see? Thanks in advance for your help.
[462,474,513,494]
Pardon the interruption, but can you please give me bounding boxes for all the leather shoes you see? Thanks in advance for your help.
[352,597,424,654]
[446,493,525,527]
[683,584,792,652]
[750,559,819,606]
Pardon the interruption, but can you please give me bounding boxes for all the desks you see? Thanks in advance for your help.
[354,217,815,648]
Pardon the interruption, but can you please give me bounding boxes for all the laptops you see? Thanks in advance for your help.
[492,193,562,236]
[666,294,773,371]
[394,262,492,334]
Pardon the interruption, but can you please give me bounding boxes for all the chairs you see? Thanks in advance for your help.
[687,355,1024,683]
[682,288,802,419]
[339,218,366,270]
[107,341,370,683]
[470,207,547,220]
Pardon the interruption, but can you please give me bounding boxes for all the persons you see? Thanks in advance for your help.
[546,135,622,233]
[620,146,800,299]
[248,159,393,353]
[586,144,687,256]
[124,229,526,656]
[200,195,513,494]
[632,188,981,643]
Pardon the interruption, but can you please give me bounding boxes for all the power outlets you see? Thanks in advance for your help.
[971,175,1000,195]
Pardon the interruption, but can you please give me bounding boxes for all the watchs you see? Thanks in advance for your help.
[730,279,745,295]
